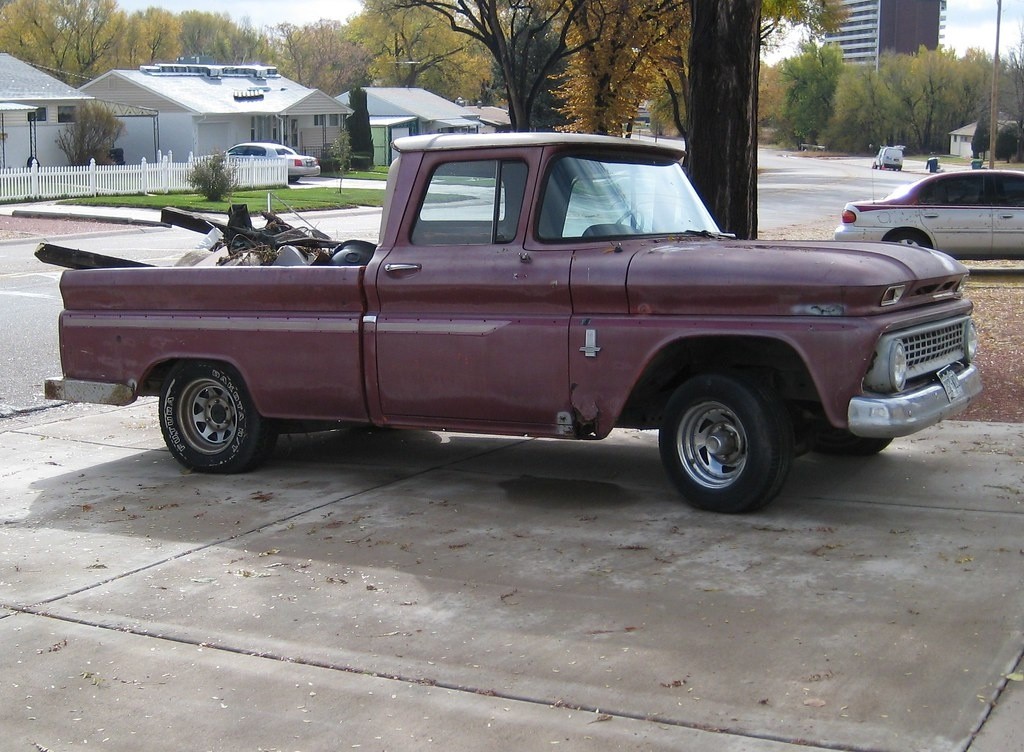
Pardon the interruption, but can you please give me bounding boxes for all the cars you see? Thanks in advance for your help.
[193,142,321,184]
[834,169,1024,261]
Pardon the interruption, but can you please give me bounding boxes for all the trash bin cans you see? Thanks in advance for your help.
[926,159,938,173]
[971,160,983,170]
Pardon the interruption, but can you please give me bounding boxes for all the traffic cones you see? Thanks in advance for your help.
[881,164,885,170]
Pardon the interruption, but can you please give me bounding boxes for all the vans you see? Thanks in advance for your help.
[872,147,903,171]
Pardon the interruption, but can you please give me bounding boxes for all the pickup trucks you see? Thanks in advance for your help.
[45,131,986,516]
